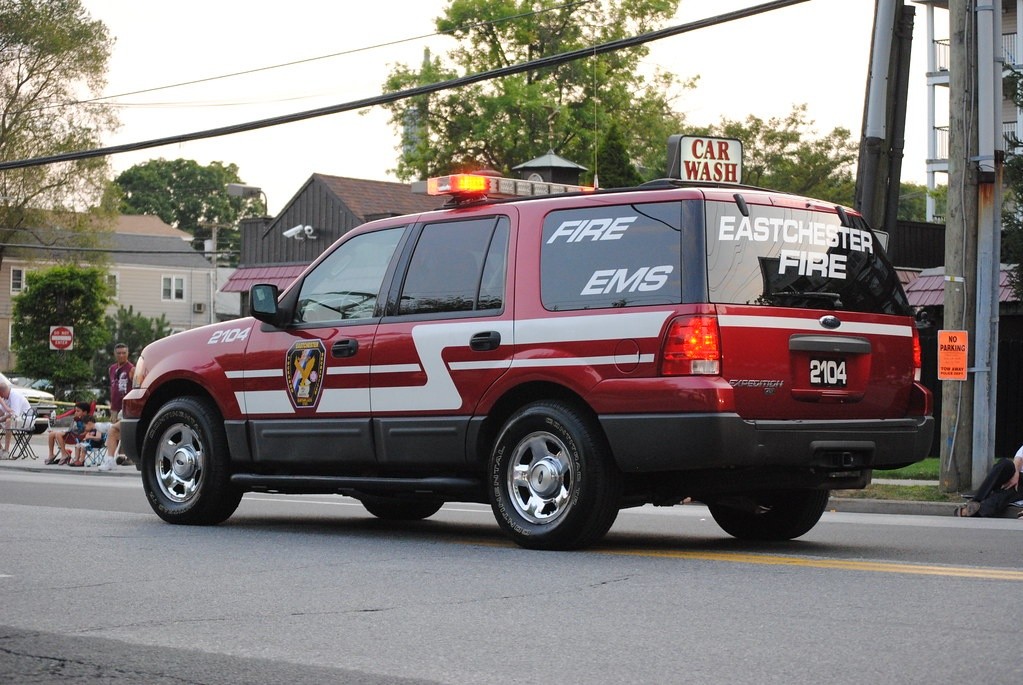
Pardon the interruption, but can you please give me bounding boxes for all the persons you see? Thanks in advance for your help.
[953,446,1023,516]
[45,343,136,471]
[0,382,35,459]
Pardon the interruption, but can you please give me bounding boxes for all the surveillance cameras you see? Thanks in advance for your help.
[305,225,313,235]
[283,224,303,238]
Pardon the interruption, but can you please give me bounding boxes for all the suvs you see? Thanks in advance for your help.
[119,168,936,551]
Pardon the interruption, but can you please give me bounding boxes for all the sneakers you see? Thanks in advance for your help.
[98,459,117,471]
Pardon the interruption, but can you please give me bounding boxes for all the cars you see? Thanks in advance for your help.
[0,373,58,434]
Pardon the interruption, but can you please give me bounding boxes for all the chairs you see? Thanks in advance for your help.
[73,432,108,467]
[5,407,38,460]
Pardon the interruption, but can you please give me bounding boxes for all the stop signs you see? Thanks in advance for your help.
[49,325,74,351]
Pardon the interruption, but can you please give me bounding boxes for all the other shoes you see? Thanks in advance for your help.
[0,448,13,460]
[68,462,74,466]
[73,461,84,466]
[116,456,125,464]
[58,455,71,465]
[954,502,980,517]
[122,458,135,465]
[45,456,56,465]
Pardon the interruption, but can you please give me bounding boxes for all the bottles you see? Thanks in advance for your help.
[51,410,56,427]
[6,413,17,428]
[94,408,106,418]
